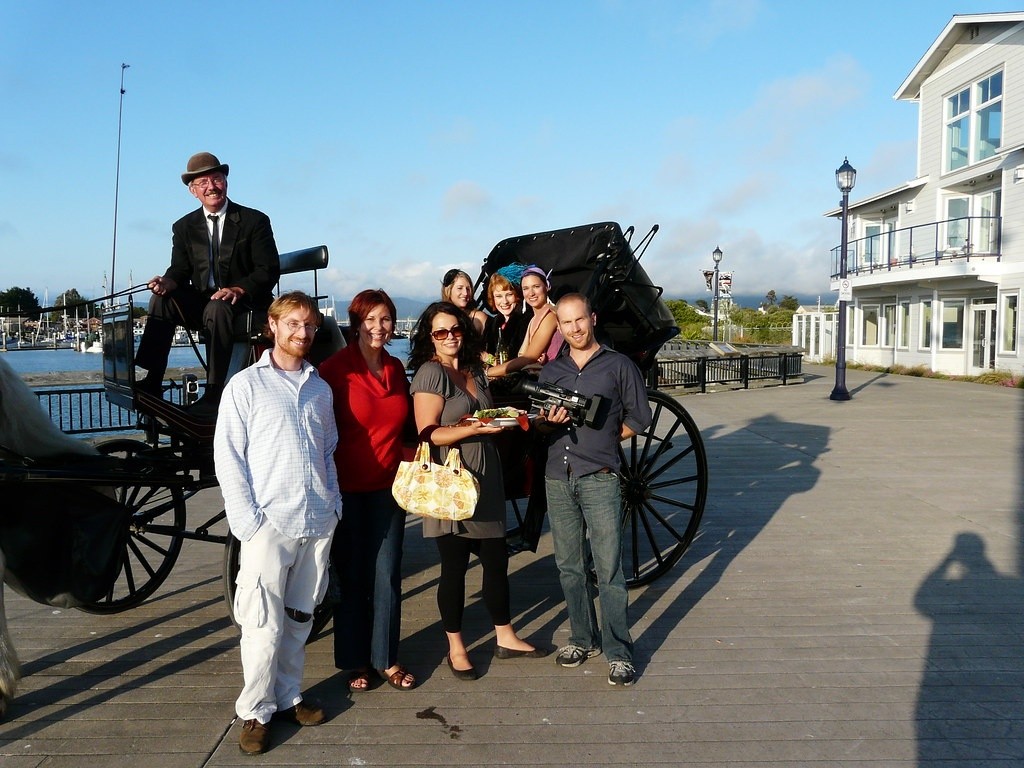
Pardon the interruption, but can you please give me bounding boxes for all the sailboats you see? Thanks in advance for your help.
[0,271,420,353]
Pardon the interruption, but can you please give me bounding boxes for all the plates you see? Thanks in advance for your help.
[466,414,538,427]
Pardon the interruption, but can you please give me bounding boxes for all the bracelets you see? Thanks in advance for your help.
[485,369,488,377]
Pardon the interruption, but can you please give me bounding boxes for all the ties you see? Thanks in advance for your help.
[207,214,220,290]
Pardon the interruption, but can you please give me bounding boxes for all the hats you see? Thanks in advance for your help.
[182,152,229,186]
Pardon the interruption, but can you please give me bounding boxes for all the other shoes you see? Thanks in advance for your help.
[137,378,163,399]
[177,397,219,416]
[447,649,476,681]
[493,642,548,659]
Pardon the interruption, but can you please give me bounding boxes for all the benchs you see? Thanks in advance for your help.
[232,245,347,343]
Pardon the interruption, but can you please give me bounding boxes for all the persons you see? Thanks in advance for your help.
[213,290,342,757]
[315,289,418,694]
[134,151,281,429]
[481,266,559,375]
[440,269,487,336]
[530,293,652,686]
[406,301,547,682]
[473,261,535,380]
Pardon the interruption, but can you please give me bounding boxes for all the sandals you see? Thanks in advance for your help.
[348,669,368,694]
[378,666,416,692]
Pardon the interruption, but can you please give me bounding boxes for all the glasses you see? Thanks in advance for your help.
[278,316,320,333]
[191,176,226,188]
[429,325,465,341]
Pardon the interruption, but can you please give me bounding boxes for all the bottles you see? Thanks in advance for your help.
[495,327,509,365]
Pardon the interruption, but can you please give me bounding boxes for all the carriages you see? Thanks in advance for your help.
[0,220,710,719]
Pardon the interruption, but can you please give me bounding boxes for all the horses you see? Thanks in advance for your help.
[1,355,99,721]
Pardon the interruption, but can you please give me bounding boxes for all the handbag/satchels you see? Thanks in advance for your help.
[392,427,481,521]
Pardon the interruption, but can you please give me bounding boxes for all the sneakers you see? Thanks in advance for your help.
[555,640,603,668]
[608,657,634,686]
[239,717,270,755]
[274,704,327,726]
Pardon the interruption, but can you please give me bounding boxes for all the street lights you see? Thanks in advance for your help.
[110,60,130,308]
[829,154,857,401]
[713,246,722,341]
